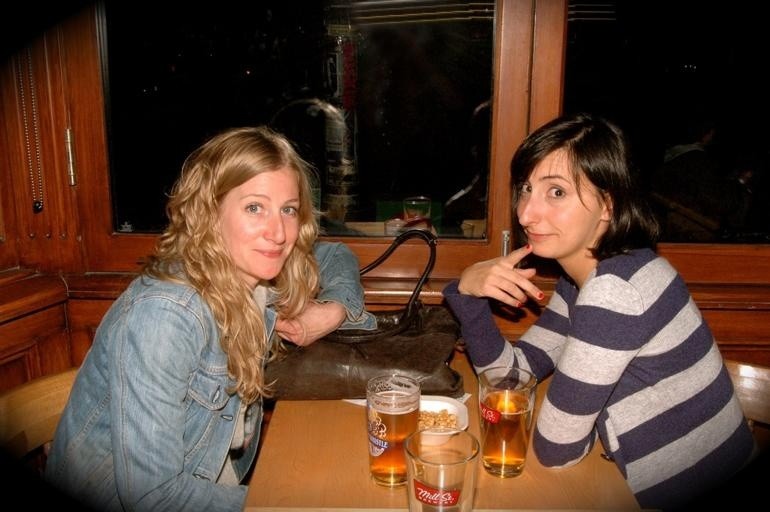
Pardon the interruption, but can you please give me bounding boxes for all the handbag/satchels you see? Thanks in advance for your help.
[263,229,465,401]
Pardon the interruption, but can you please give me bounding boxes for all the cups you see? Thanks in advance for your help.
[404,426,481,512]
[479,366,537,478]
[404,196,431,223]
[384,218,408,237]
[366,374,420,488]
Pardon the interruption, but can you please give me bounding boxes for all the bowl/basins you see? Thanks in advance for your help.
[419,395,469,446]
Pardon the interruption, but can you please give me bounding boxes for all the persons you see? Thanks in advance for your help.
[269,98,349,233]
[443,113,758,512]
[44,126,378,512]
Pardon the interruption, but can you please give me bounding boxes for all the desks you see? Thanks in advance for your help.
[240,345,641,511]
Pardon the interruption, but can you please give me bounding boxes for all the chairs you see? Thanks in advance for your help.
[720,359,770,446]
[1,364,77,491]
